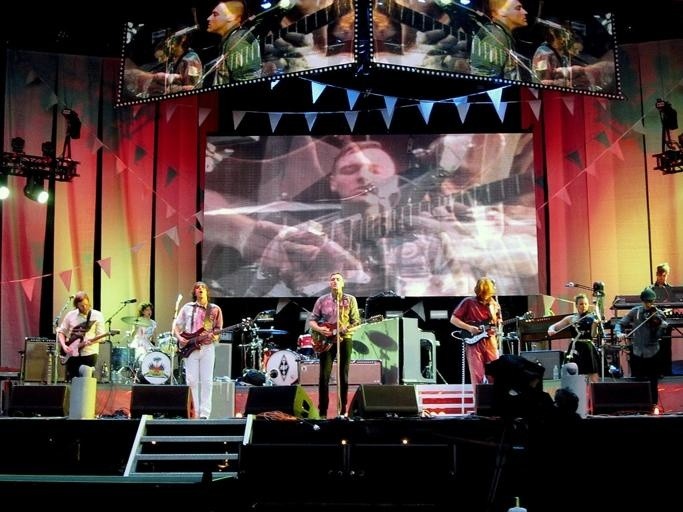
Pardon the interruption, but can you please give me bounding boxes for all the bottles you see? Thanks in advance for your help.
[553,365,559,380]
[268,375,272,387]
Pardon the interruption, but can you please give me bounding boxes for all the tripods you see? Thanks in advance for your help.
[115,342,141,383]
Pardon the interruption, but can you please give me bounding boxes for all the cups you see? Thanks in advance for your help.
[508,507,527,512]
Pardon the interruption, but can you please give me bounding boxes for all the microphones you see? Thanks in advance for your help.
[316,184,376,202]
[564,282,574,288]
[336,283,339,293]
[70,295,75,298]
[441,0,484,16]
[173,24,199,36]
[176,294,183,304]
[389,290,405,299]
[121,299,137,303]
[248,0,290,19]
[536,18,564,30]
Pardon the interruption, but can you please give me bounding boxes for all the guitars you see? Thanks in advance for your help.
[177,317,253,357]
[201,159,476,285]
[203,168,535,298]
[461,311,534,346]
[61,330,120,365]
[311,314,384,352]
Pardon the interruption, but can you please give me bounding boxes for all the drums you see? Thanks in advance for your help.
[266,350,300,384]
[134,351,171,384]
[112,346,135,372]
[155,332,178,354]
[299,334,314,355]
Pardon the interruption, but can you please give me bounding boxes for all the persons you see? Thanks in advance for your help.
[614,288,668,415]
[308,273,361,422]
[649,263,672,376]
[548,293,602,382]
[450,277,504,416]
[128,302,157,348]
[203,134,538,298]
[123,0,263,97]
[173,282,223,419]
[470,0,615,89]
[58,291,105,382]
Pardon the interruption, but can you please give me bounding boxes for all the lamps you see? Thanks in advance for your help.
[23,176,49,205]
[0,173,10,200]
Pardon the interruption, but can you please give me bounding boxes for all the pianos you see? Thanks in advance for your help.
[604,296,683,328]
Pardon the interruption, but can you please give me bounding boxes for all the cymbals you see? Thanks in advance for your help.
[122,316,152,327]
[256,329,288,335]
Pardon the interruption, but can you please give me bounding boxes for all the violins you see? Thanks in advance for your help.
[581,311,600,327]
[643,307,674,330]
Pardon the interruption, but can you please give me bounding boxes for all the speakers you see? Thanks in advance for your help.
[9,386,70,416]
[475,384,493,416]
[213,342,232,380]
[348,384,418,420]
[198,382,235,419]
[520,351,564,379]
[299,362,382,385]
[244,385,320,420]
[93,339,112,384]
[130,384,195,418]
[590,377,652,415]
[23,337,67,382]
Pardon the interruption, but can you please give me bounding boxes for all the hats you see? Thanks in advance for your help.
[641,288,656,300]
